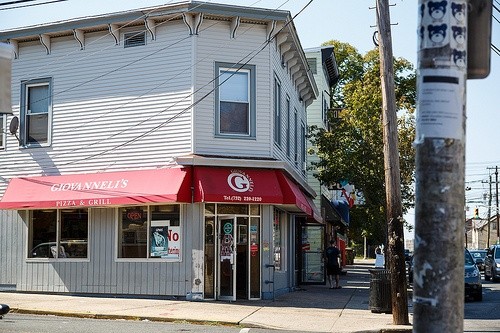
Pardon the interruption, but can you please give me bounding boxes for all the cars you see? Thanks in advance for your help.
[31,240,87,259]
[405,248,489,301]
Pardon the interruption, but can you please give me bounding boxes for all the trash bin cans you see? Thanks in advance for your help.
[367,267,393,314]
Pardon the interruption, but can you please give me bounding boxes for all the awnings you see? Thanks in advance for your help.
[193,164,314,215]
[0,167,191,212]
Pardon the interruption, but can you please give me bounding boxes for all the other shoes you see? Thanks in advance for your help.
[336,286,342,289]
[329,287,335,289]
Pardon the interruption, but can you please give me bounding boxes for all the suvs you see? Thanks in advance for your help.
[483,245,500,281]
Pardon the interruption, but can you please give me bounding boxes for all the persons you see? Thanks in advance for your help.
[374,246,385,268]
[322,239,343,289]
